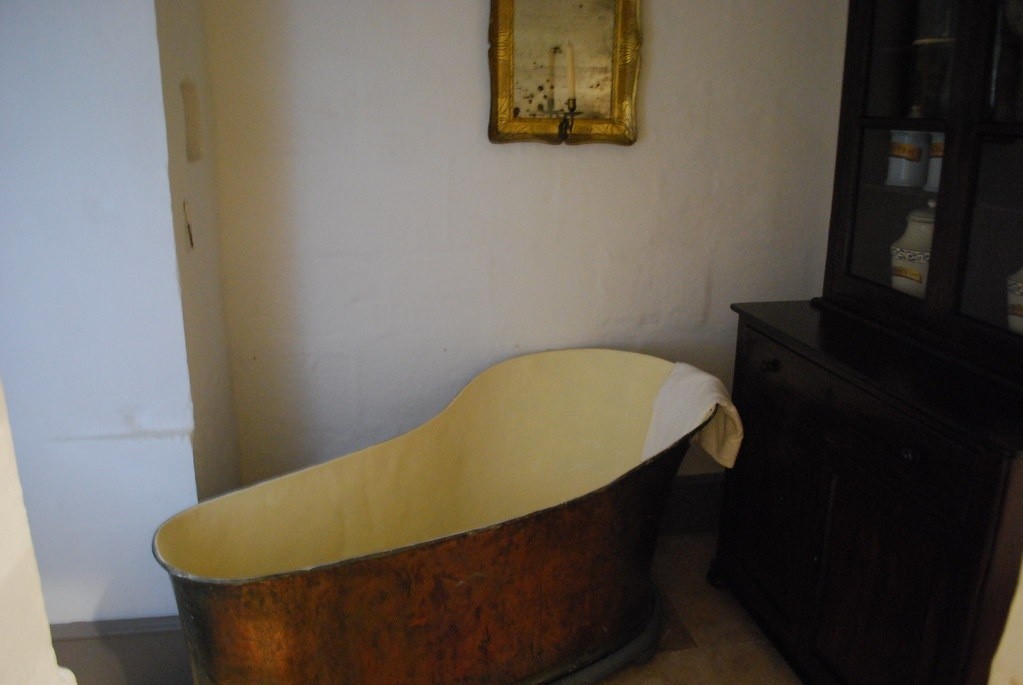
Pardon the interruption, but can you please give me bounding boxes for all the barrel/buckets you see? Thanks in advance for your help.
[883,130,930,187]
[924,132,945,192]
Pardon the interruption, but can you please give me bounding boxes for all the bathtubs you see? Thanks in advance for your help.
[148,346,721,684]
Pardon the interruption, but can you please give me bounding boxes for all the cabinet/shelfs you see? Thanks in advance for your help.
[704,302,1022,685]
[810,0,1023,393]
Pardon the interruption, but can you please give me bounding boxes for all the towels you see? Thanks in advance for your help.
[642,361,745,470]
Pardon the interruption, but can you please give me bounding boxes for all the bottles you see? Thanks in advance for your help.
[1006,266,1023,336]
[890,207,935,298]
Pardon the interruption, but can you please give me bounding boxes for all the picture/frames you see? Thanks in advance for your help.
[487,0,643,147]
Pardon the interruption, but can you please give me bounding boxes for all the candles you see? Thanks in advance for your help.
[567,42,576,98]
